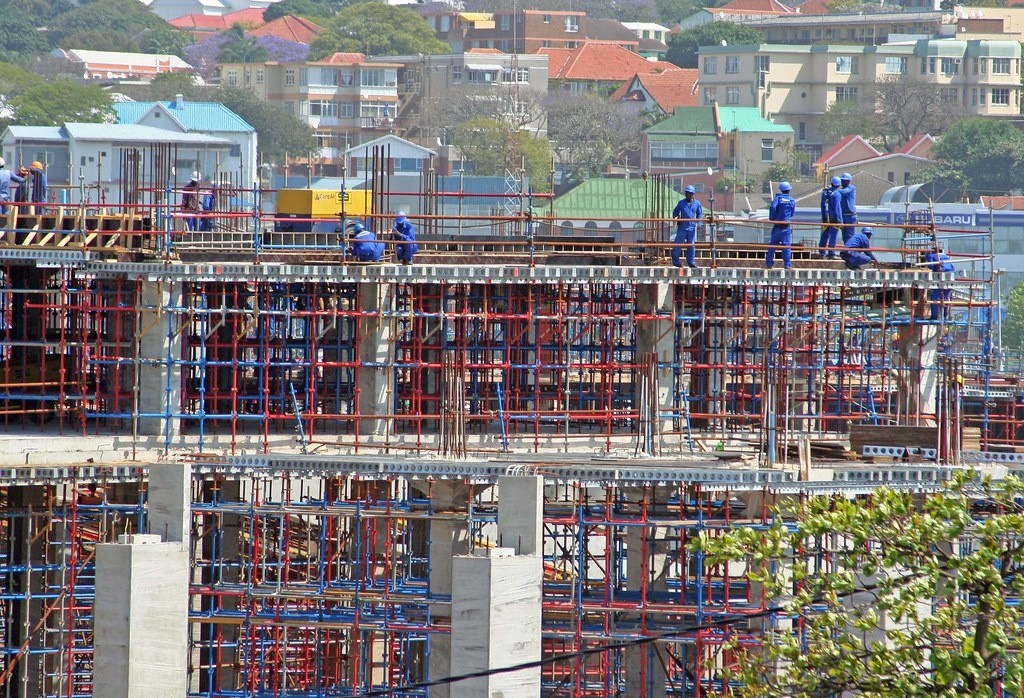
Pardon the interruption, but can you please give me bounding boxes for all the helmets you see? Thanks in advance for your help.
[354,223,365,233]
[395,211,406,224]
[831,176,841,187]
[0,157,5,166]
[29,160,44,170]
[861,227,873,233]
[840,172,852,181]
[779,182,792,191]
[191,171,202,182]
[684,185,695,194]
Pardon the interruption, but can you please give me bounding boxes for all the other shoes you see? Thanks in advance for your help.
[689,264,698,268]
[829,255,842,260]
[402,259,407,265]
[673,262,683,267]
[926,315,939,320]
[407,260,413,265]
[845,262,856,270]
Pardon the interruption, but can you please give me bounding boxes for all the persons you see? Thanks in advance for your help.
[392,212,418,264]
[840,227,879,270]
[985,330,994,371]
[181,171,201,230]
[820,177,844,260]
[352,224,386,262]
[847,332,856,362]
[926,248,954,319]
[29,161,46,215]
[200,182,217,231]
[943,328,953,353]
[15,168,29,214]
[766,182,795,269]
[0,157,27,215]
[672,185,702,268]
[837,172,858,250]
